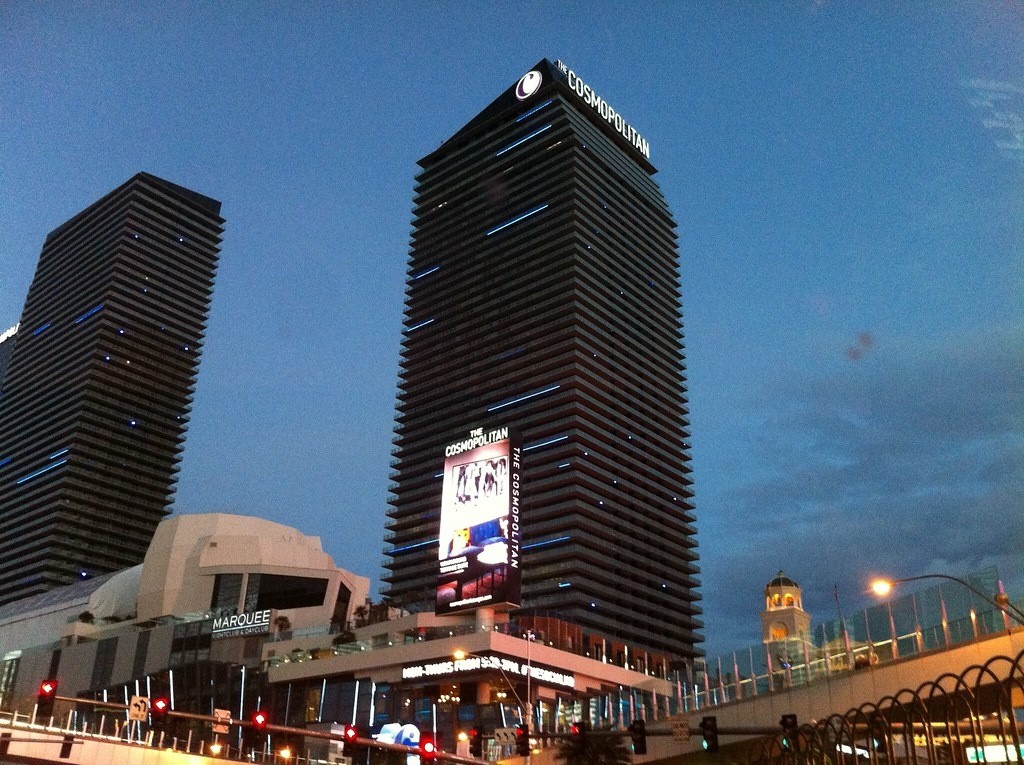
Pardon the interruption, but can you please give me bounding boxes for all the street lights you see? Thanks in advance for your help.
[454,628,531,733]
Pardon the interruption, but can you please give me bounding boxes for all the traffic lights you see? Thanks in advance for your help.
[627,719,647,755]
[468,725,483,757]
[698,716,719,754]
[344,724,358,742]
[514,723,529,757]
[150,694,170,718]
[779,713,801,754]
[37,677,59,701]
[420,737,436,758]
[571,721,586,742]
[250,711,269,733]
